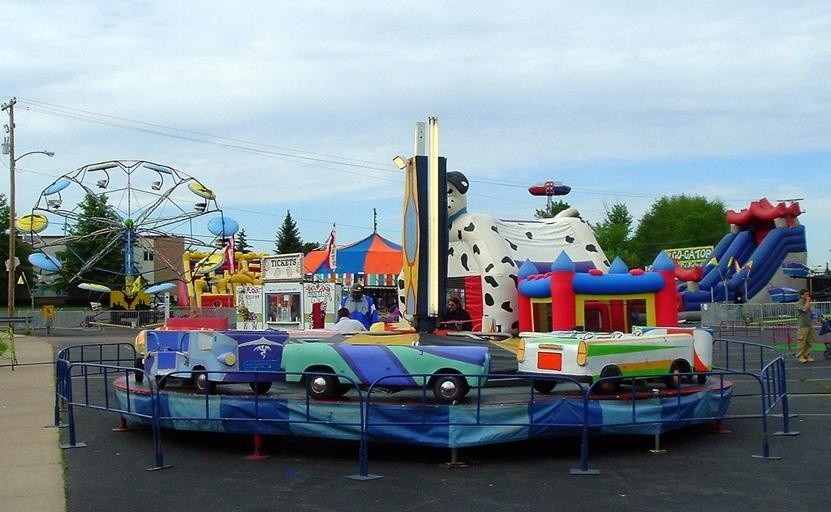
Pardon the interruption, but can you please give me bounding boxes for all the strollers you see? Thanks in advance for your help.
[813,312,831,360]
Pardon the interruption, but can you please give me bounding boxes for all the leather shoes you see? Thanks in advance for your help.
[798,355,815,363]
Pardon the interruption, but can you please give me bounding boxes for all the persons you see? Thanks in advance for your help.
[795,288,816,364]
[134,299,146,310]
[443,297,473,331]
[333,284,378,331]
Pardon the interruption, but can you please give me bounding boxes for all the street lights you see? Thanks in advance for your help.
[6,143,55,332]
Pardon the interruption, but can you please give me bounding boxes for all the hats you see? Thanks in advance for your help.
[350,283,363,292]
[338,307,350,318]
[448,292,461,303]
